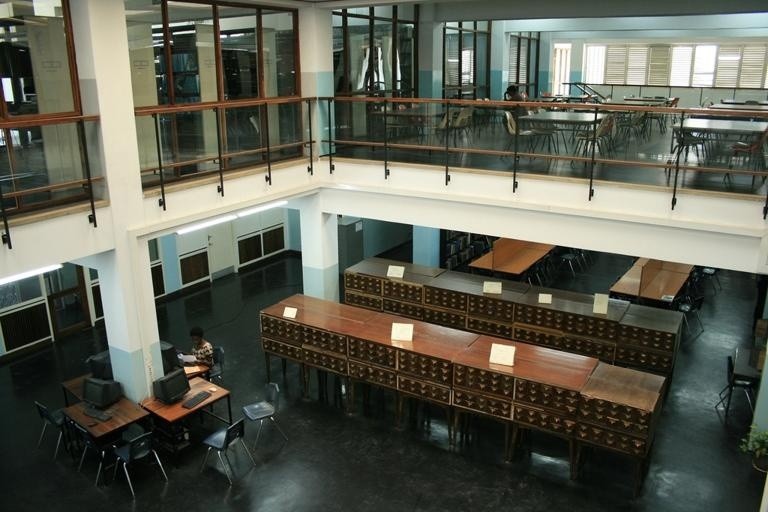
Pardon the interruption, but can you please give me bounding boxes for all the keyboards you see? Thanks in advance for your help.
[182,391,212,409]
[83,409,112,421]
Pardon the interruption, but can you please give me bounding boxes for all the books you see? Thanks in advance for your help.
[178,353,197,364]
[183,366,201,374]
[225,109,261,138]
[158,51,202,117]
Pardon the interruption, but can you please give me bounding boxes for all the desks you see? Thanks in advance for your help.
[63,397,149,440]
[60,374,94,402]
[139,376,233,466]
[469,236,694,303]
[184,360,210,378]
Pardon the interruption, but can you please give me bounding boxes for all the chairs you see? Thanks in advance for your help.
[714,354,755,428]
[365,76,768,193]
[70,418,125,487]
[677,267,723,336]
[205,345,225,384]
[111,431,169,495]
[34,400,71,460]
[242,382,289,450]
[200,416,256,486]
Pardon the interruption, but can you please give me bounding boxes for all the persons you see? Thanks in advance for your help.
[504,85,531,132]
[174,325,216,377]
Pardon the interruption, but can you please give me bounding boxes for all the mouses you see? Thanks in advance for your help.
[88,422,98,426]
[209,387,217,392]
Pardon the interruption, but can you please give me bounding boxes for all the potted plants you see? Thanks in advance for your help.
[739,430,768,486]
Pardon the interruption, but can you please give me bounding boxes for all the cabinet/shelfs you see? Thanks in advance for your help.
[615,304,683,384]
[512,284,629,364]
[258,293,378,410]
[346,312,482,442]
[452,334,598,479]
[574,362,668,499]
[423,270,531,340]
[343,257,445,321]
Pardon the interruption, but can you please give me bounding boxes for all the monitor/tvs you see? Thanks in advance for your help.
[153,368,190,404]
[81,378,122,408]
[160,341,180,375]
[89,350,113,379]
